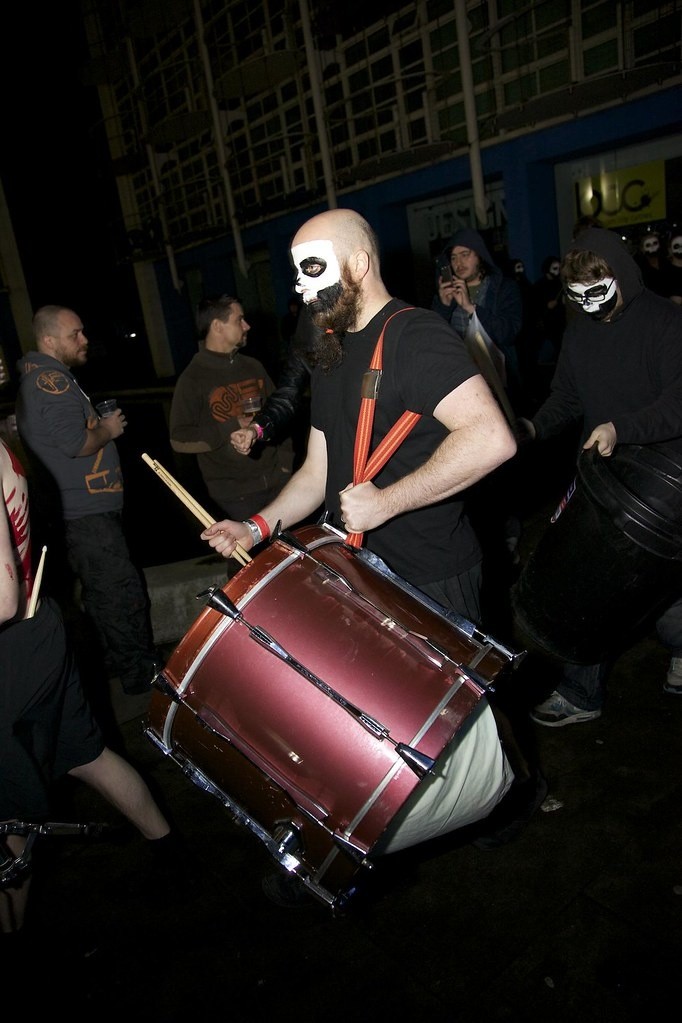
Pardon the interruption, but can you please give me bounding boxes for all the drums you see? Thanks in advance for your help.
[135,510,530,911]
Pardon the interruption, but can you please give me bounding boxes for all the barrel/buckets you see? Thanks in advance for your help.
[511,441,682,666]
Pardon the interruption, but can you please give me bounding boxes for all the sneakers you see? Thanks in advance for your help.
[529,691,601,725]
[663,656,682,695]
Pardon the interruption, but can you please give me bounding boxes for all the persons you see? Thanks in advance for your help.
[0,437,210,934]
[15,305,180,695]
[169,216,682,728]
[200,207,549,848]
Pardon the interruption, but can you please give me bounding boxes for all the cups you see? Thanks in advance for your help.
[242,397,261,417]
[95,400,117,417]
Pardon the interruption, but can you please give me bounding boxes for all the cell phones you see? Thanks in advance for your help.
[441,265,453,288]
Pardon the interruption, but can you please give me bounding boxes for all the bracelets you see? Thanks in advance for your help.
[249,423,263,439]
[243,514,270,547]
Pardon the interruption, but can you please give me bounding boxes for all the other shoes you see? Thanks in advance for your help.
[98,832,176,880]
[114,643,173,695]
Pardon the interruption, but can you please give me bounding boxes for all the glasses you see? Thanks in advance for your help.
[565,277,617,302]
[448,250,478,265]
